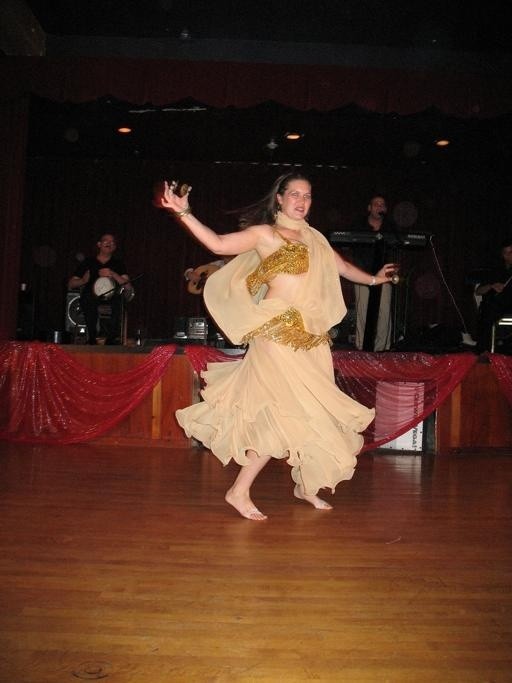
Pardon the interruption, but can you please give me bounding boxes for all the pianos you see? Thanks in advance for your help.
[330,229,427,244]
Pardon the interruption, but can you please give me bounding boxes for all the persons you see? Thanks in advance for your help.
[344,191,404,352]
[469,240,511,355]
[160,171,399,520]
[182,254,237,348]
[69,232,133,344]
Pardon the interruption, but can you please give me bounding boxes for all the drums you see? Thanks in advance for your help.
[93,276,118,298]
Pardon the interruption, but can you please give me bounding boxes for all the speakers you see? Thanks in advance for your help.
[64,289,101,335]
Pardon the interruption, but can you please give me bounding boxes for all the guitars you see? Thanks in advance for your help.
[187,265,220,295]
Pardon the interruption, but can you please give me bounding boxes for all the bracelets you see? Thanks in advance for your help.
[172,206,192,217]
[369,275,376,285]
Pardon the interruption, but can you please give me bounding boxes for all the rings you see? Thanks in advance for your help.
[164,190,168,195]
[186,189,190,193]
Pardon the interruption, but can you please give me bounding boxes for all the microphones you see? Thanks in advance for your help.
[378,210,387,221]
